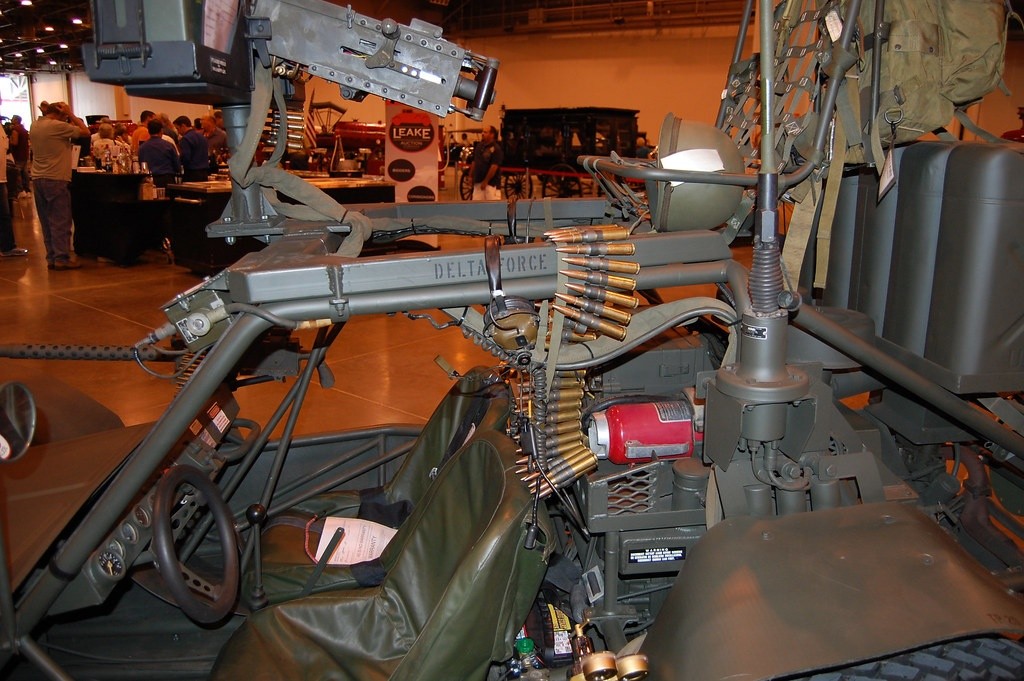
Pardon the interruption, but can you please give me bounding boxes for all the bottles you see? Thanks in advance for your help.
[504,124,564,159]
[445,123,474,170]
[79,141,149,174]
[209,147,231,167]
[278,140,384,177]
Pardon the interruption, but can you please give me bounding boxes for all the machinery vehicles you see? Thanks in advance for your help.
[0,1,1024,681]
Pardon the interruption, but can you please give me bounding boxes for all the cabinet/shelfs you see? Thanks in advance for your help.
[71,168,166,267]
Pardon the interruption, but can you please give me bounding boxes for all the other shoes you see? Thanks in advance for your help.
[1,248,29,257]
[18,191,26,199]
[26,192,32,198]
[55,260,81,270]
[48,262,55,270]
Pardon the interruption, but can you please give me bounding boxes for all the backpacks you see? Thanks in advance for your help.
[720,0,1024,293]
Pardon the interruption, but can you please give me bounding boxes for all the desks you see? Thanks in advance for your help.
[167,178,397,277]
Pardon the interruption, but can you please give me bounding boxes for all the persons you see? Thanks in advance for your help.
[72,118,90,159]
[539,137,560,170]
[636,138,653,159]
[91,118,137,162]
[138,119,182,189]
[30,101,91,269]
[173,116,209,182]
[456,134,472,148]
[468,126,503,200]
[131,110,155,155]
[156,112,180,156]
[0,115,30,256]
[194,111,227,148]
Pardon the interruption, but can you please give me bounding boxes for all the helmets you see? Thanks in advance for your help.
[644,111,745,233]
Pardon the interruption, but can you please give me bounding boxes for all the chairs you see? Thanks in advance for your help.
[237,364,516,608]
[209,423,553,681]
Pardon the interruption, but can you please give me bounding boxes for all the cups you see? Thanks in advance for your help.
[157,187,165,199]
[207,168,231,181]
